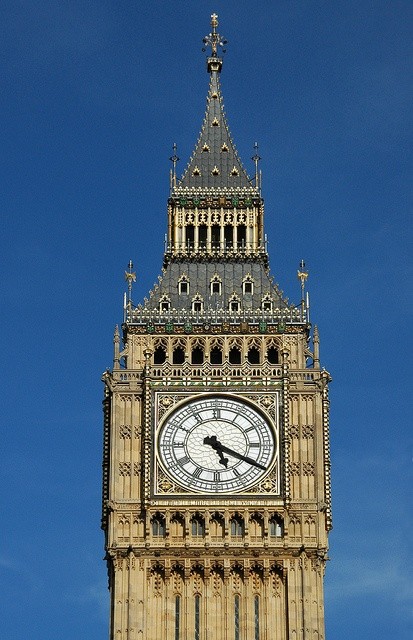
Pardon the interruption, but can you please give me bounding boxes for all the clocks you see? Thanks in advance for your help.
[154,394,279,494]
